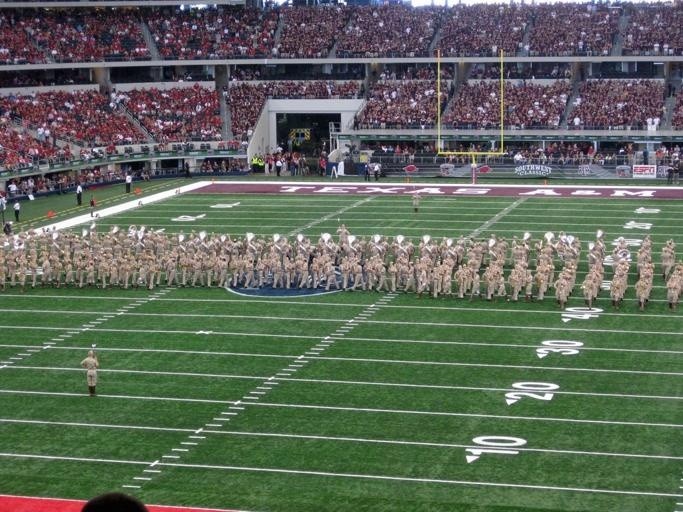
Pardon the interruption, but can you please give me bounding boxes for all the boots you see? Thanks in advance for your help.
[88,386,96,398]
[0,284,676,312]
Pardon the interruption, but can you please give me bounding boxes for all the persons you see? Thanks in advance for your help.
[0,191,7,226]
[90,195,96,217]
[76,181,82,205]
[81,492,149,512]
[80,350,99,397]
[13,200,20,221]
